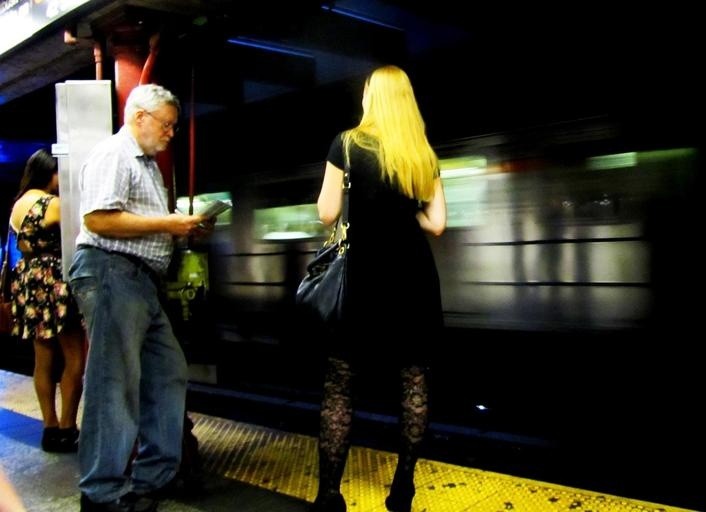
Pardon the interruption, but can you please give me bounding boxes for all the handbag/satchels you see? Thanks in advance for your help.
[1,261,11,303]
[295,240,347,319]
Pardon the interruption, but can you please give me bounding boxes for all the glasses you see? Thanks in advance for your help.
[136,103,180,133]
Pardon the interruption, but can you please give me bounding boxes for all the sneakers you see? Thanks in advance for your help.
[80,493,159,512]
[42,428,80,452]
[309,495,347,511]
[386,484,415,511]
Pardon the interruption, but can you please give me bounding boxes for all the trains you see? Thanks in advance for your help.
[154,82,704,456]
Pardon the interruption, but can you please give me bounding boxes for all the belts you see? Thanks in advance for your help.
[76,245,161,285]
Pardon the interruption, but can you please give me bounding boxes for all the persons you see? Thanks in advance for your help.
[309,62,449,510]
[68,81,215,512]
[10,147,89,450]
